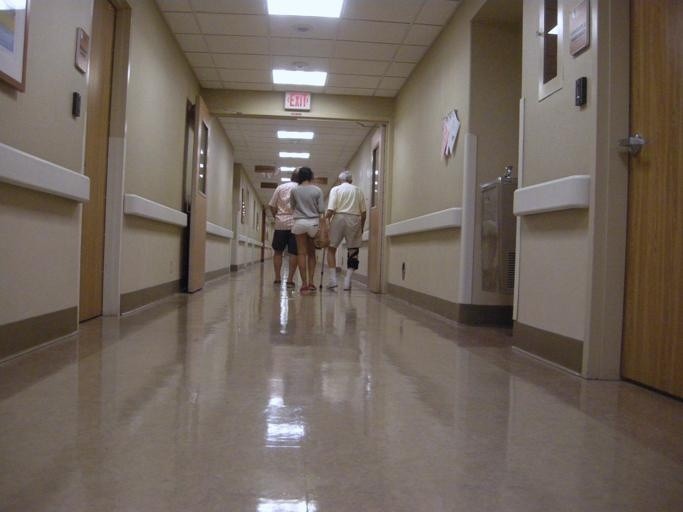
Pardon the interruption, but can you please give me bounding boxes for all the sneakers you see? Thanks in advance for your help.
[273,279,280,286]
[286,281,295,288]
[300,284,317,291]
[326,280,338,290]
[343,283,352,291]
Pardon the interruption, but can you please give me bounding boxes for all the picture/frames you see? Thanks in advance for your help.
[0,2,34,94]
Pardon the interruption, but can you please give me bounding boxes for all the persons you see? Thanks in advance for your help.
[320,169,368,291]
[266,167,302,289]
[288,166,325,295]
